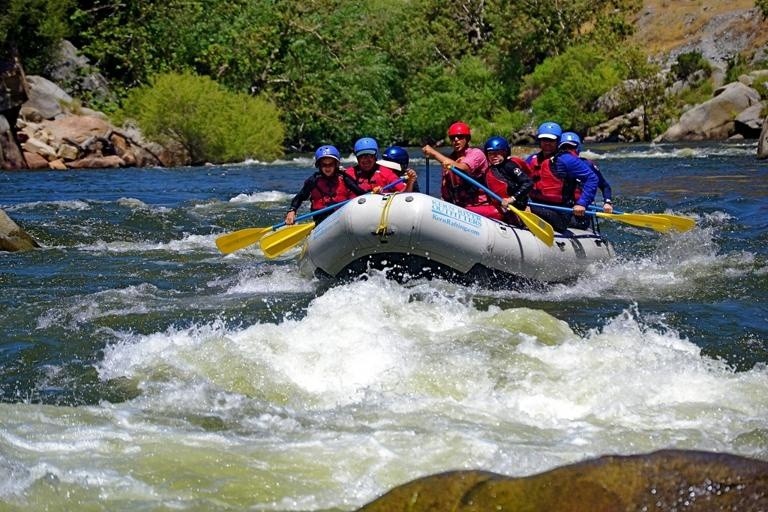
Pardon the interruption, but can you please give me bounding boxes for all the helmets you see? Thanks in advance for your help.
[448,122,581,156]
[316,137,409,175]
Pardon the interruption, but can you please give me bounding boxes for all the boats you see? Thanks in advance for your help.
[307,188,614,289]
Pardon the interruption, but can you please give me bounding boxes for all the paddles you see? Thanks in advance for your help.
[588,204,695,233]
[444,161,553,246]
[259,174,409,259]
[215,191,373,254]
[526,202,672,233]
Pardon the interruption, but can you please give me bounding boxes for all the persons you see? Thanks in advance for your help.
[526,121,599,236]
[465,137,534,229]
[423,122,492,210]
[343,136,417,197]
[284,146,387,227]
[558,132,613,231]
[382,146,421,192]
